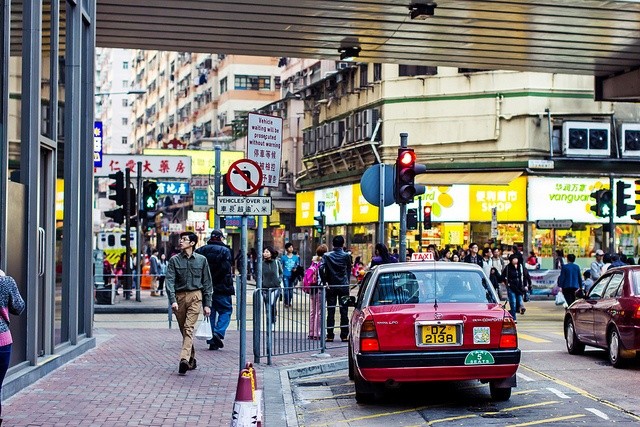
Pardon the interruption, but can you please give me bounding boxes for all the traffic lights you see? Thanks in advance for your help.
[313,216,323,232]
[108,171,123,205]
[104,207,124,224]
[143,181,157,209]
[597,189,611,216]
[414,160,426,195]
[590,192,597,211]
[424,205,431,229]
[398,148,415,204]
[623,183,635,215]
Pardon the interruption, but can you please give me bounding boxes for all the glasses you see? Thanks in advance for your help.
[181,237,190,241]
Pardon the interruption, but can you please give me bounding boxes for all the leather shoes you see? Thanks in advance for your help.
[213,333,224,348]
[209,342,219,350]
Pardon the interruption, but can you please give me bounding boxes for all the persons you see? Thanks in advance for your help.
[151,250,159,293]
[504,254,529,322]
[262,246,283,332]
[319,236,352,341]
[493,249,506,297]
[357,264,365,283]
[166,232,213,375]
[589,249,603,279]
[556,249,564,266]
[355,257,363,276]
[616,246,627,261]
[629,258,637,266]
[512,247,523,262]
[558,253,583,306]
[483,246,493,276]
[281,243,299,307]
[1,268,25,427]
[526,250,537,265]
[465,243,485,267]
[459,250,466,262]
[371,241,397,265]
[427,245,437,259]
[104,255,114,290]
[159,253,167,293]
[608,254,626,269]
[391,249,400,263]
[195,230,235,350]
[440,249,451,262]
[583,272,593,291]
[302,246,328,340]
[237,247,258,280]
[116,252,130,295]
[453,253,461,262]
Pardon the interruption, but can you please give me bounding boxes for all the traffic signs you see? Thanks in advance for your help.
[216,195,272,214]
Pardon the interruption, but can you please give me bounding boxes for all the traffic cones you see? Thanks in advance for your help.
[246,361,262,426]
[232,368,257,426]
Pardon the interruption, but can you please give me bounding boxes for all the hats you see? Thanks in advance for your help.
[595,249,605,256]
[211,229,225,238]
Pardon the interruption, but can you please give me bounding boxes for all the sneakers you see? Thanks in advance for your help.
[179,360,188,374]
[308,336,314,339]
[188,358,197,370]
[325,337,333,341]
[342,336,349,341]
[313,337,321,339]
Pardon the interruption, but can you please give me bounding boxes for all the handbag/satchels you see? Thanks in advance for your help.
[523,291,531,303]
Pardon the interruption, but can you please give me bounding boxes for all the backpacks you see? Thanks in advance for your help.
[303,263,322,294]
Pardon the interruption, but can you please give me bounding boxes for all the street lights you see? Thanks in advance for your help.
[95,89,148,98]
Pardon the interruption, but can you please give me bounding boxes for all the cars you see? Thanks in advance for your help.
[564,264,640,367]
[93,259,118,304]
[347,252,522,406]
